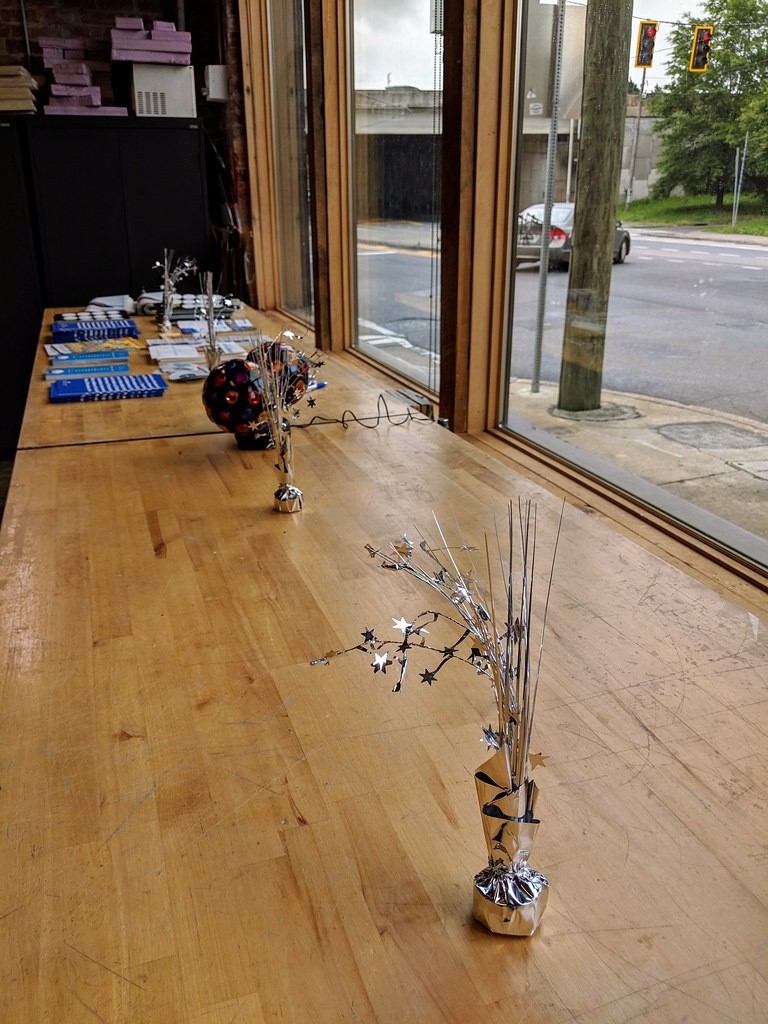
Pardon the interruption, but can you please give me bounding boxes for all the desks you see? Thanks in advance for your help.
[0,296,768,1024]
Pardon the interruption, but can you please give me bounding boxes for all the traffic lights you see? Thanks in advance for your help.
[689,26,713,72]
[635,20,659,68]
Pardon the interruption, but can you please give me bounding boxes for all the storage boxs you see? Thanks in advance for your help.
[39,16,193,114]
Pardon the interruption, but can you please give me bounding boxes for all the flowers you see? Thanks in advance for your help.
[306,491,571,791]
[246,332,318,447]
[154,247,198,314]
[201,271,230,349]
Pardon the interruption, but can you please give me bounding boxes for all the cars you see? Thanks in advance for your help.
[516,203,631,273]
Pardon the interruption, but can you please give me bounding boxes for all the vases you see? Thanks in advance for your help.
[469,743,548,937]
[159,313,172,332]
[204,349,222,370]
[264,436,304,513]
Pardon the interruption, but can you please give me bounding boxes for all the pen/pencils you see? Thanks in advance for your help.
[158,308,233,326]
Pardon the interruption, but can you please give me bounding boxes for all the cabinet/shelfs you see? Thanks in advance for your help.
[0,114,219,462]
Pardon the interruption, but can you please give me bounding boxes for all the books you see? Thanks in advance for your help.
[0,65,42,116]
